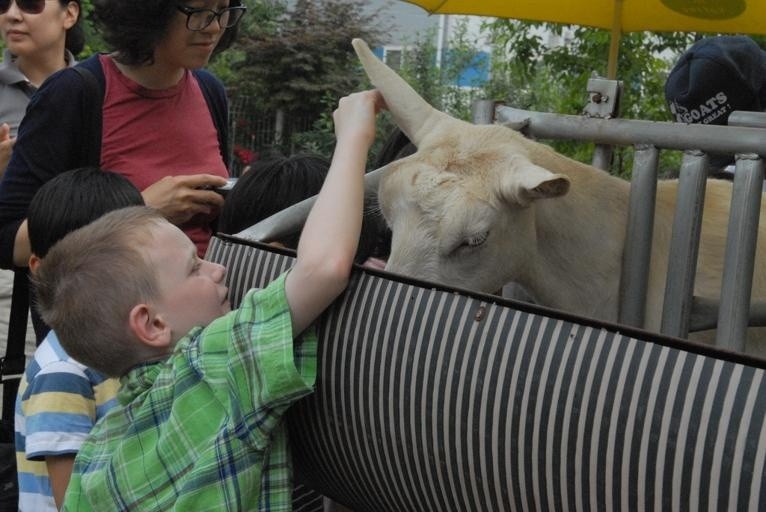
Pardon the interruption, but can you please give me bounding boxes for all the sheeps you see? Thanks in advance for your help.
[351,39,766,360]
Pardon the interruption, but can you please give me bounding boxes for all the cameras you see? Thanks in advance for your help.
[196,177,240,228]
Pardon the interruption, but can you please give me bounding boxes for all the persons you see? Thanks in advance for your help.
[13,165,150,512]
[1,1,251,349]
[27,85,391,511]
[213,147,386,270]
[0,0,85,179]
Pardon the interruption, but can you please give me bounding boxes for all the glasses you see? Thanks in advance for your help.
[176,2,248,31]
[0,0,45,14]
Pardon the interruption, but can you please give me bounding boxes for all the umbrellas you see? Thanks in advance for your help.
[400,1,766,81]
